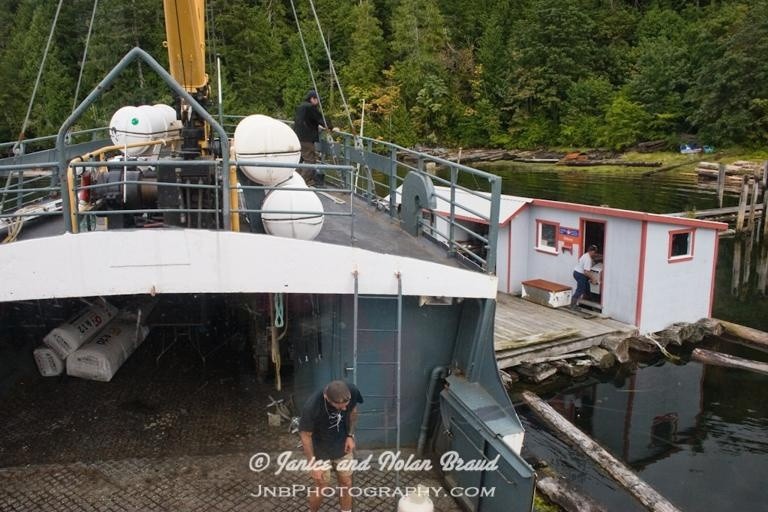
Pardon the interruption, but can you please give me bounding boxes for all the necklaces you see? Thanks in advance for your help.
[325,400,340,419]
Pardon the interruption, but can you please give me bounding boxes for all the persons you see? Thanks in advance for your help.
[295,91,341,187]
[568,244,597,316]
[299,381,364,512]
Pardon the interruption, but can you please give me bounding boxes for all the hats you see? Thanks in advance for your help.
[302,90,318,101]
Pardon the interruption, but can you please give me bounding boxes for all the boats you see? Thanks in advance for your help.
[0,0,538,512]
